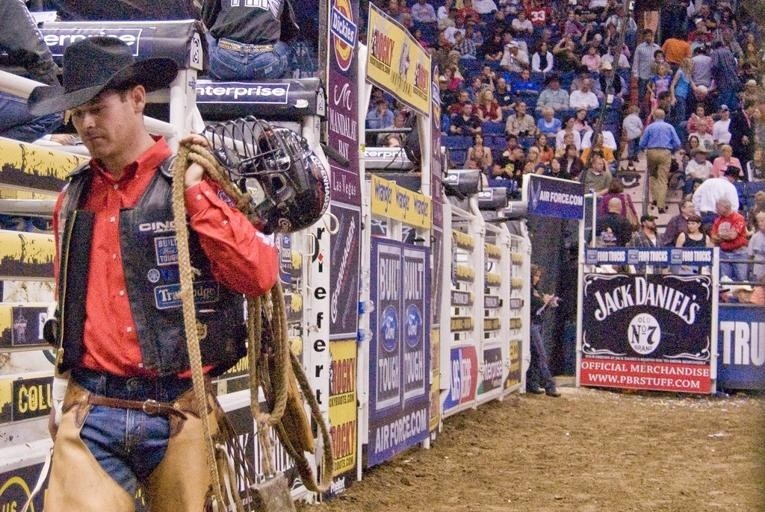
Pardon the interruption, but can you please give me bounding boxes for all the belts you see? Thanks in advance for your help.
[219,41,274,54]
[87,394,190,423]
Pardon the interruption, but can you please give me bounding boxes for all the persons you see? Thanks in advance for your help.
[360,0,765,305]
[525,262,562,397]
[198,0,302,84]
[22,37,284,511]
[0,1,68,144]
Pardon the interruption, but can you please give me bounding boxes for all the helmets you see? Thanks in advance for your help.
[200,115,330,237]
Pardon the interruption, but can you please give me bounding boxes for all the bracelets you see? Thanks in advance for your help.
[50,377,68,400]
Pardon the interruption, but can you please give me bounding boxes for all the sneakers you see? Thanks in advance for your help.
[527,387,545,394]
[546,390,561,397]
[652,200,665,213]
[628,156,637,166]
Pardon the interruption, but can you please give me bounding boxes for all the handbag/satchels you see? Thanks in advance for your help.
[626,206,640,233]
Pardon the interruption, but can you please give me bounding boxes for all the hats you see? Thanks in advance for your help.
[719,283,730,293]
[577,65,592,74]
[439,75,448,83]
[511,144,528,151]
[745,79,757,87]
[25,36,180,119]
[733,280,754,296]
[600,63,613,71]
[640,215,658,221]
[720,105,730,113]
[720,166,746,181]
[690,145,712,156]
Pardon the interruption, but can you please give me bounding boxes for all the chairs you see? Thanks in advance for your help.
[434,14,764,232]
